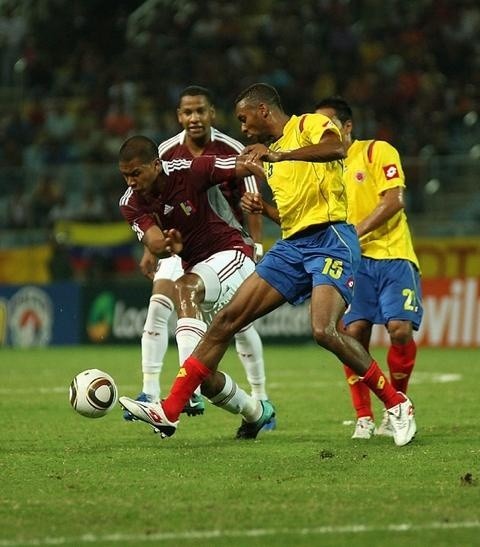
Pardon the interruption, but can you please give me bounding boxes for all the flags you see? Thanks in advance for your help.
[53,219,137,276]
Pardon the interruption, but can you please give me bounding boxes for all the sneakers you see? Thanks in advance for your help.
[118,390,205,439]
[234,400,276,439]
[351,391,416,447]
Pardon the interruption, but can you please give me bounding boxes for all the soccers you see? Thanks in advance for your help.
[69,370,117,420]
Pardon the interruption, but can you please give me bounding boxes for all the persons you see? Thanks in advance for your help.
[117,84,418,448]
[309,95,424,443]
[116,84,280,434]
[117,134,275,442]
[2,2,480,229]
